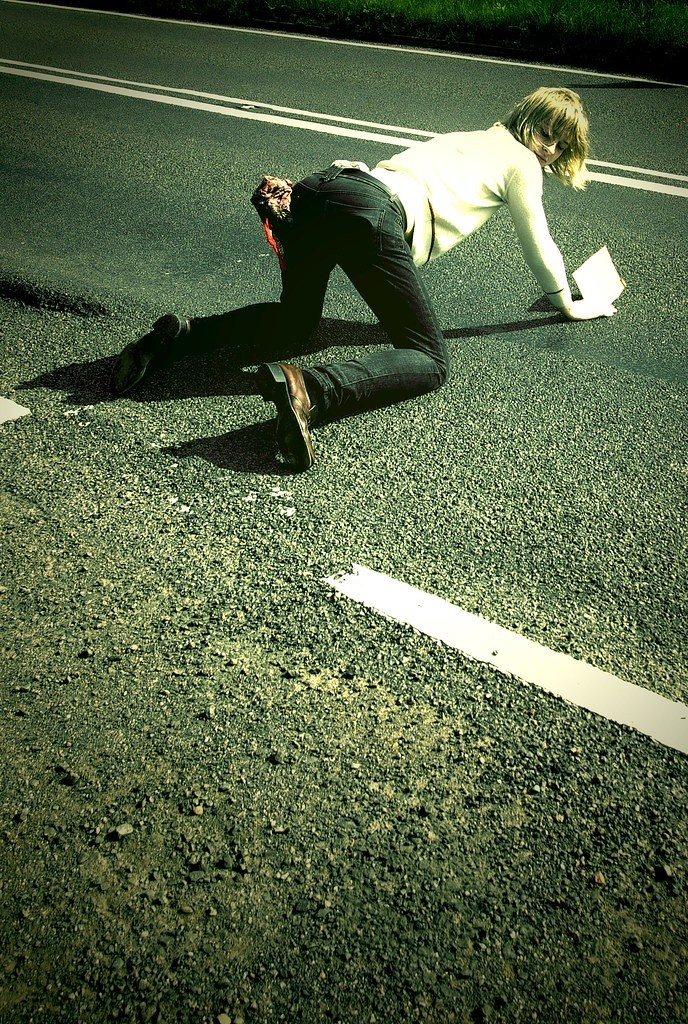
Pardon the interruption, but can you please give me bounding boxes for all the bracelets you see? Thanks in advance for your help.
[542,288,564,295]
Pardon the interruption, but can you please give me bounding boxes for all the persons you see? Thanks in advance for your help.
[110,85,618,471]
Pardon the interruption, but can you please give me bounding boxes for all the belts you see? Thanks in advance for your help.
[323,165,407,230]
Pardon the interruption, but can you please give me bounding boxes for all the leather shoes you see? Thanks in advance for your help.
[108,314,190,397]
[259,361,317,469]
